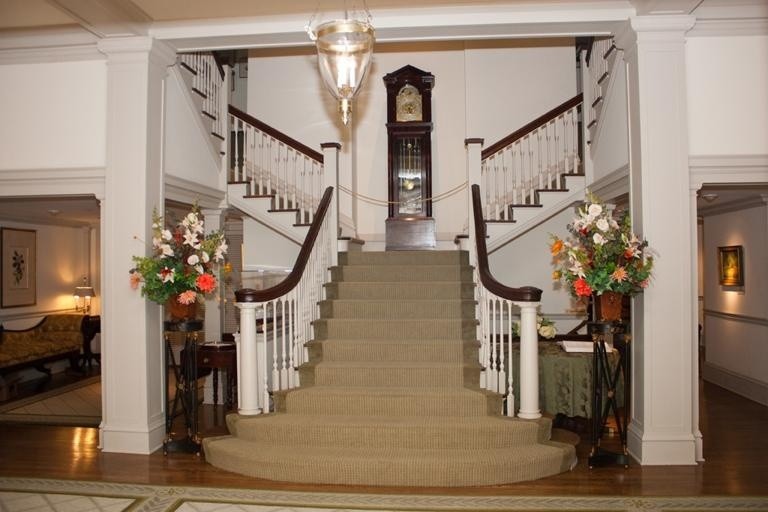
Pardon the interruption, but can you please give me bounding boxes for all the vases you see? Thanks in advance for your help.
[167,295,197,319]
[592,290,625,322]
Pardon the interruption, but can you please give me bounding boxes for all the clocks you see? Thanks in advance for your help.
[382,64,435,250]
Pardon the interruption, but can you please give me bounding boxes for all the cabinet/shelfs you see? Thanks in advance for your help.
[491,341,623,419]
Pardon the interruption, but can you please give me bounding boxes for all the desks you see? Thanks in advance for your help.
[77,316,100,368]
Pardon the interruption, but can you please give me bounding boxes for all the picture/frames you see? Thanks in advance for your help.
[0,228,36,308]
[717,246,744,286]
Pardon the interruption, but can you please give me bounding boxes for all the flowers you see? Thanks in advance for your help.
[128,196,237,307]
[545,186,660,308]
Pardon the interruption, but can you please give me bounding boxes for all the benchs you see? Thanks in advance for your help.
[0,313,92,378]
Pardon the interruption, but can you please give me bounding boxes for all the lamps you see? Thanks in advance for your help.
[306,0,375,126]
[73,277,96,314]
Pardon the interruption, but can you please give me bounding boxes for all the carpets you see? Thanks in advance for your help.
[0,476,768,512]
[201,250,579,487]
[0,368,205,427]
